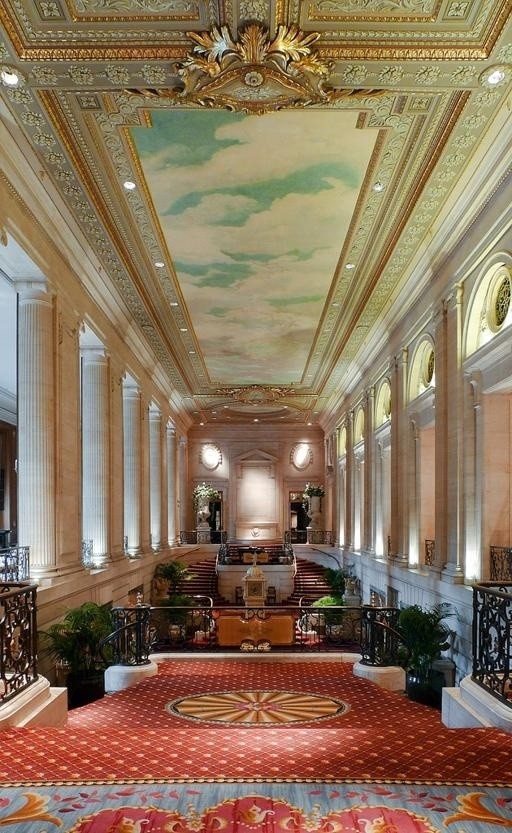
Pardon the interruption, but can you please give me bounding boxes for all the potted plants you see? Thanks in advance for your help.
[308,594,346,641]
[154,560,199,595]
[303,481,327,526]
[190,481,223,526]
[38,599,122,711]
[159,595,198,640]
[398,601,464,708]
